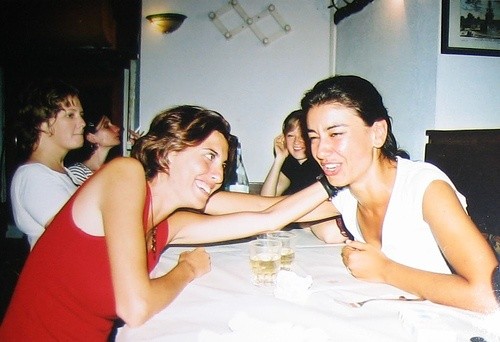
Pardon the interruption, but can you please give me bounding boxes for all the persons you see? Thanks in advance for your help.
[11,80,86,252]
[69,107,121,185]
[260,110,351,244]
[0,106,336,341]
[128,75,498,314]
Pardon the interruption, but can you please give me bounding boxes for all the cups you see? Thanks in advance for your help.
[247,239,283,286]
[267,230,295,269]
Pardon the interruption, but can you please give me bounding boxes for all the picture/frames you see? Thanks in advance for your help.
[440,0,500,57]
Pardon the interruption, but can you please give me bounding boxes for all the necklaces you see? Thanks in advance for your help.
[150,192,158,261]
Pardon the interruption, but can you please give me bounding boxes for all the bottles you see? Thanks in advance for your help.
[228,143,249,194]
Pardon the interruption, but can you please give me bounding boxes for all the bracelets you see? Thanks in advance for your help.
[319,176,336,199]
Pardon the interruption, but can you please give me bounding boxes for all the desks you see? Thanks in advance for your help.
[114,224,500,342]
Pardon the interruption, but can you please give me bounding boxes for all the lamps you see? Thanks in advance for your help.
[146,12,187,34]
